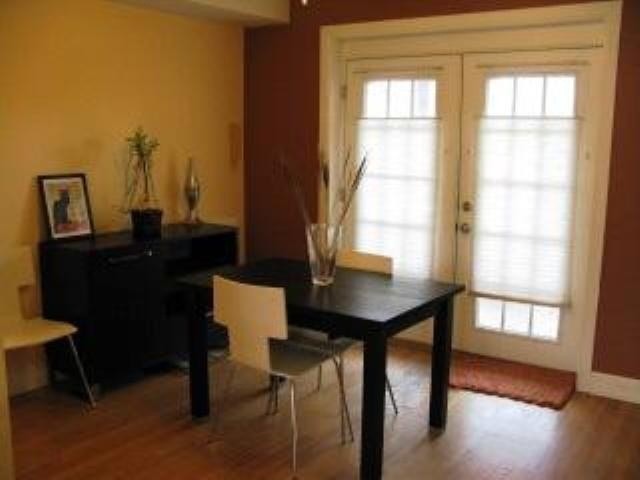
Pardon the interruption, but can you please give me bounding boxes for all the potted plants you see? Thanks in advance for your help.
[117,126,164,240]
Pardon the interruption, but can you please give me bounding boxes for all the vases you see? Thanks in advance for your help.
[303,224,344,286]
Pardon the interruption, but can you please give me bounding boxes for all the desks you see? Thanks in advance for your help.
[180,256,465,480]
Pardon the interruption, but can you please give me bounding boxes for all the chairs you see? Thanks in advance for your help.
[0,245,97,411]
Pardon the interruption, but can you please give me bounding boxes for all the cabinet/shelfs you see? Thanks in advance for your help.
[37,220,239,400]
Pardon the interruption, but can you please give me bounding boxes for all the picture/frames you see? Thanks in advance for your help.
[35,172,96,243]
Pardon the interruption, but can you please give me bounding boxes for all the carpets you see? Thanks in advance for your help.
[450,349,577,411]
